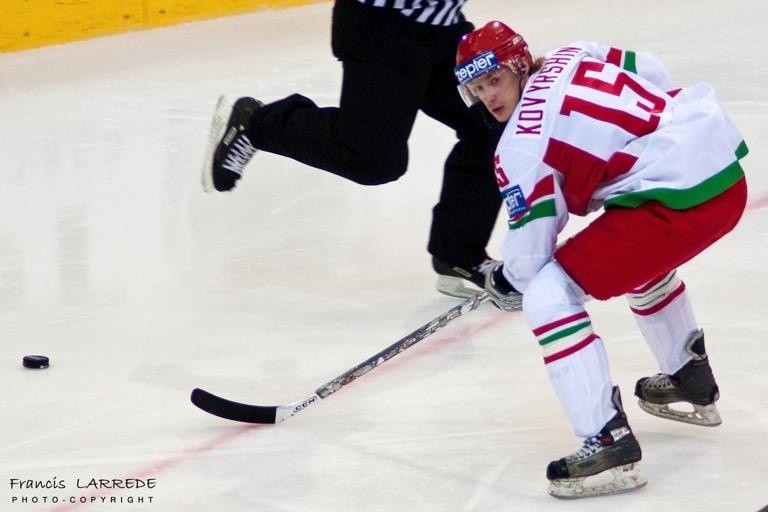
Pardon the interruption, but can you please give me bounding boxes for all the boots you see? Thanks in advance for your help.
[634,329,720,406]
[546,383,642,481]
[211,96,264,192]
[432,242,496,289]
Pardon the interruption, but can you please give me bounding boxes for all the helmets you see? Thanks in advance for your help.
[452,19,532,108]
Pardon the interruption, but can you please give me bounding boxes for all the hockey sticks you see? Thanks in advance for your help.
[191,291,490,424]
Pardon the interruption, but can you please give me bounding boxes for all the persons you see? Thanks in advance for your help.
[212,0,508,290]
[453,20,749,479]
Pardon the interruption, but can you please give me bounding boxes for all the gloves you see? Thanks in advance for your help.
[484,264,523,312]
